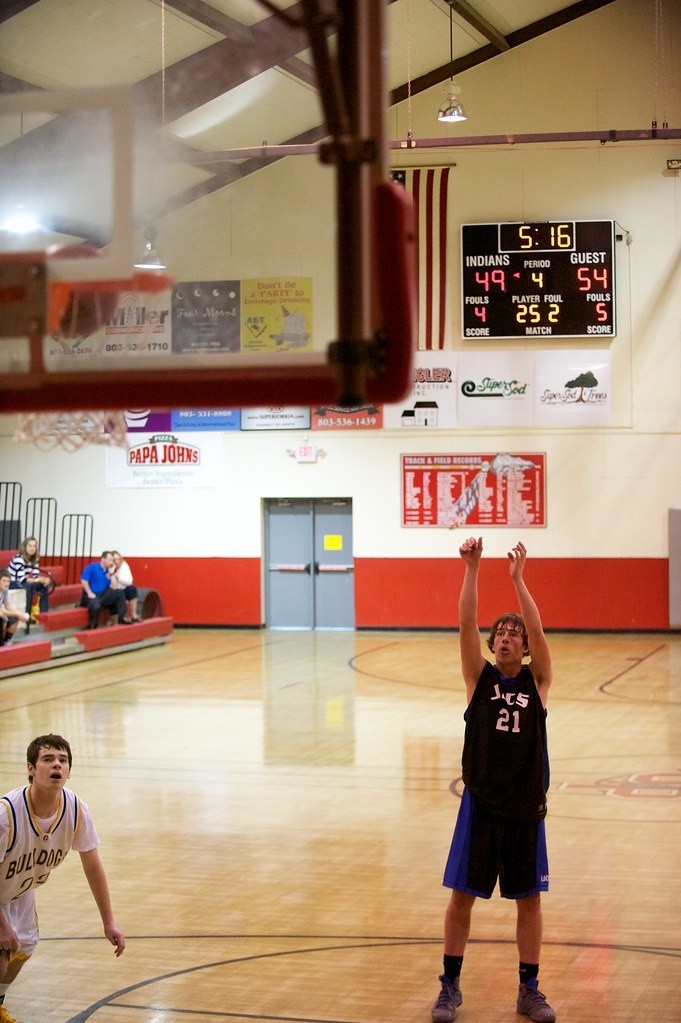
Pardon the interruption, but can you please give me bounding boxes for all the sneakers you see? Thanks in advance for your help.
[431,975,462,1023]
[517,977,555,1023]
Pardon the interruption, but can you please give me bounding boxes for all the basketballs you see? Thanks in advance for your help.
[44,242,117,337]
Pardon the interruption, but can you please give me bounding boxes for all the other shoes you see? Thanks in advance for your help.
[89,624,97,630]
[118,617,133,624]
[0,1004,16,1023]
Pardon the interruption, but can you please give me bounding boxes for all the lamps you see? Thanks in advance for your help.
[667,159,681,169]
[437,0,468,121]
[134,236,168,272]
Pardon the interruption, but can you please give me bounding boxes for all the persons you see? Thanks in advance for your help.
[0,568,29,646]
[431,535,556,1023]
[6,537,51,614]
[0,732,126,1023]
[80,551,143,629]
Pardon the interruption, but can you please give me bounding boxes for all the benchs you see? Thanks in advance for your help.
[0,549,176,680]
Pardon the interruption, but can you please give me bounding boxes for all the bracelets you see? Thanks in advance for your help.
[35,578,39,583]
[110,573,114,576]
